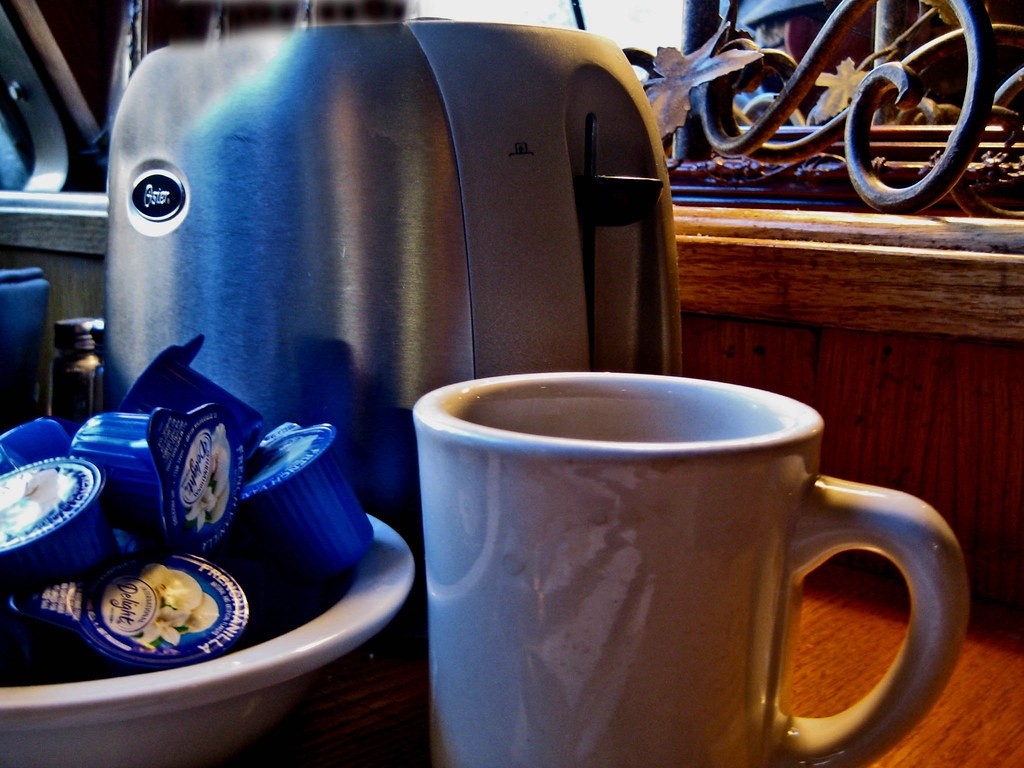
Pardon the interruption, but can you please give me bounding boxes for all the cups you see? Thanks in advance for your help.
[413,371,969,768]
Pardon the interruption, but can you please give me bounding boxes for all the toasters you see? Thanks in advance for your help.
[105,18,683,530]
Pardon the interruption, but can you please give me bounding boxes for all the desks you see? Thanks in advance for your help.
[203,559,1023,768]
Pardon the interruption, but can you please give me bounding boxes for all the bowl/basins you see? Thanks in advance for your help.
[0,512,415,768]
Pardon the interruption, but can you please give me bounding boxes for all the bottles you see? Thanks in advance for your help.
[51,317,105,424]
